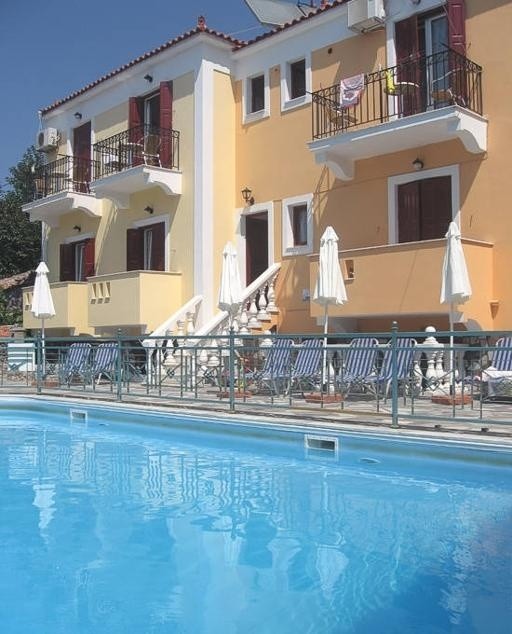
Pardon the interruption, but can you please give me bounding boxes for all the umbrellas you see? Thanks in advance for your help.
[30,261,55,374]
[312,225,348,382]
[439,221,472,385]
[217,239,246,330]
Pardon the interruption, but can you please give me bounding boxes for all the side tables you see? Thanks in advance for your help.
[383,81,418,119]
[118,143,144,167]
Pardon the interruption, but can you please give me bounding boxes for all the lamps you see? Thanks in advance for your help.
[411,157,423,171]
[241,186,255,205]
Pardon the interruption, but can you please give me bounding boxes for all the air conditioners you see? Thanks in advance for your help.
[37,128,58,153]
[346,0,386,36]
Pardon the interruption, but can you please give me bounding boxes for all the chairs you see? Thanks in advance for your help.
[126,134,163,168]
[429,67,469,109]
[324,100,358,137]
[242,337,512,418]
[33,164,89,201]
[56,343,119,393]
